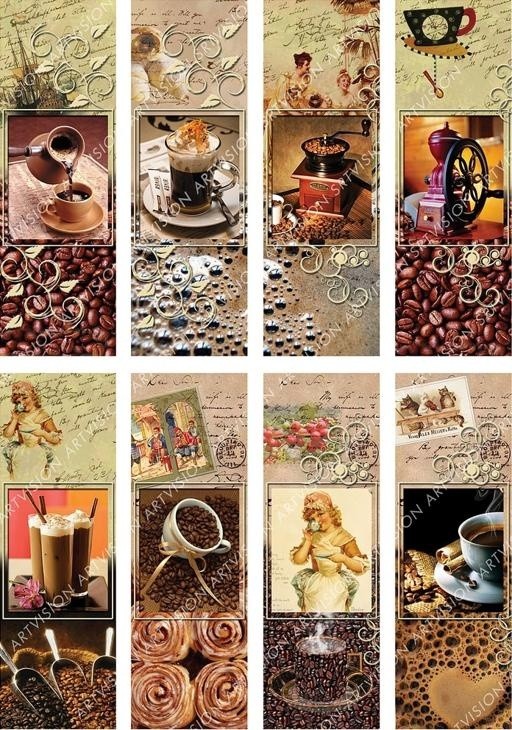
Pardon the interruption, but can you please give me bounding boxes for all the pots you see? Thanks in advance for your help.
[301,134,350,173]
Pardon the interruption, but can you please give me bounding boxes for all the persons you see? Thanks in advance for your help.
[289,491,370,613]
[2,382,62,482]
[269,52,358,115]
[148,420,203,465]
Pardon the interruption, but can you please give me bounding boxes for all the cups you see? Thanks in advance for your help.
[403,6,477,47]
[159,499,231,557]
[272,194,293,225]
[458,511,505,584]
[27,511,93,606]
[296,636,346,702]
[46,182,93,222]
[164,132,239,212]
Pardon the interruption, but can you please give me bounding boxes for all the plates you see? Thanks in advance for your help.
[143,170,241,229]
[40,200,104,233]
[434,555,505,604]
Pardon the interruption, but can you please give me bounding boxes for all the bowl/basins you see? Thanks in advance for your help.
[268,661,372,713]
